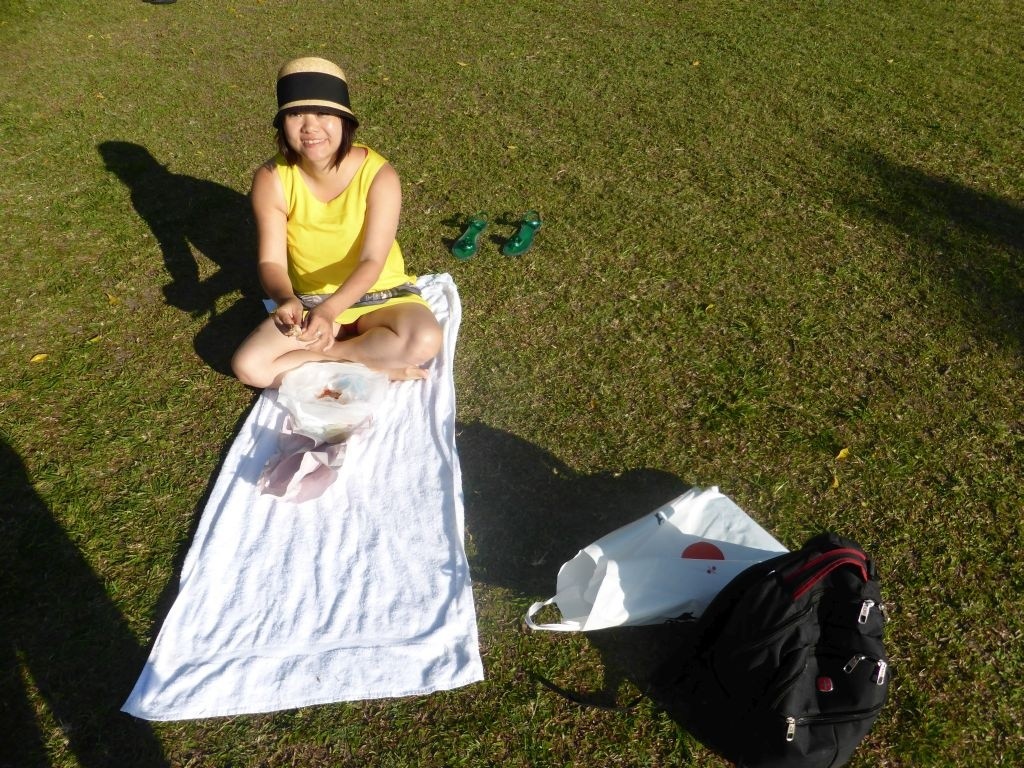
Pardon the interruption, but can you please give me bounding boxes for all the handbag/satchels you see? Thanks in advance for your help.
[524,485,789,630]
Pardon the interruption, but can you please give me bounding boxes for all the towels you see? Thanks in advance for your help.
[118,270,487,723]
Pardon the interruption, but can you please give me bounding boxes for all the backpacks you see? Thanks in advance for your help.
[686,531,889,768]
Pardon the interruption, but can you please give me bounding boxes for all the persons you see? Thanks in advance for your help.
[231,57,444,389]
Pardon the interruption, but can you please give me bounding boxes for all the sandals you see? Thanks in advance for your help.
[503,211,541,256]
[452,210,488,259]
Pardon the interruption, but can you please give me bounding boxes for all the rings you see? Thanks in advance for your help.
[316,329,322,335]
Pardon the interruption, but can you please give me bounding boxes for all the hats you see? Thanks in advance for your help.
[272,56,359,130]
